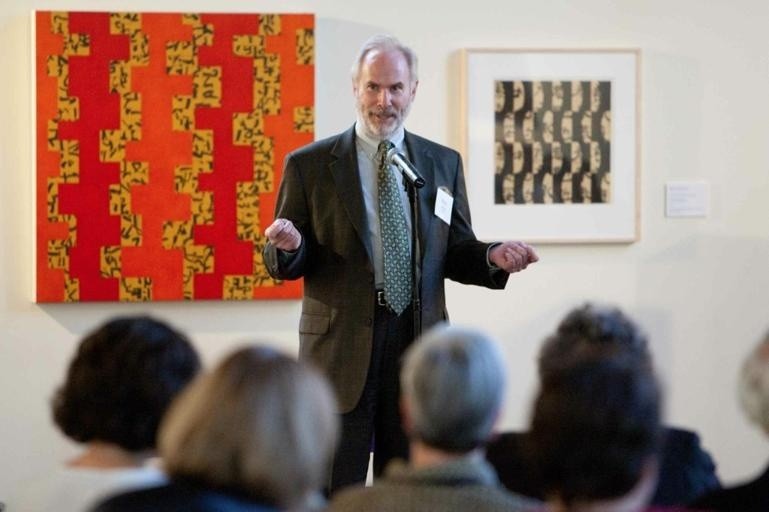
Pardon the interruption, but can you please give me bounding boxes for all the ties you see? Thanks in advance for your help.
[378,140,412,318]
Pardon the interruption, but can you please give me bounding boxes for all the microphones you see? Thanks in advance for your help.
[377,139,426,189]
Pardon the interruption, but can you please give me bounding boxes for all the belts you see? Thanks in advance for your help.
[376,289,387,310]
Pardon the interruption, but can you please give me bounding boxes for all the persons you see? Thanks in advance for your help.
[528,350,676,511]
[484,298,724,506]
[257,30,541,497]
[2,312,209,511]
[316,317,552,511]
[687,325,769,510]
[94,336,349,510]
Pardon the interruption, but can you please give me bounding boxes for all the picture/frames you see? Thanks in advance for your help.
[460,46,639,246]
